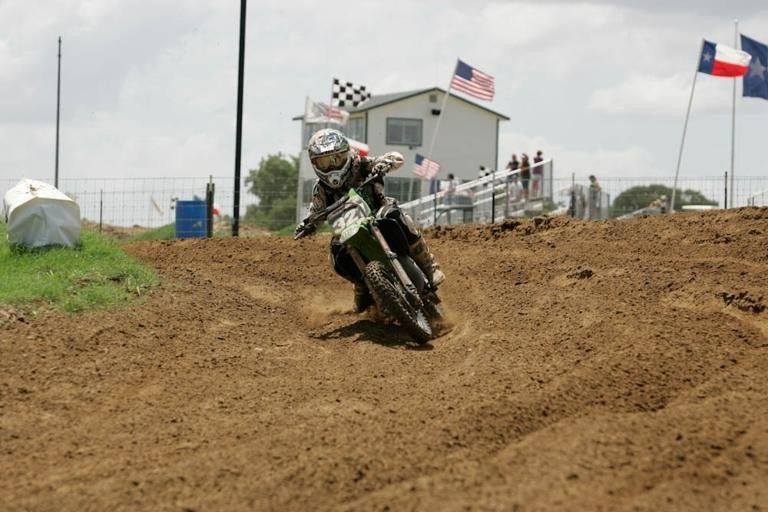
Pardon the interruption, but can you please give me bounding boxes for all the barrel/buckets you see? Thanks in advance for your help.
[175,200,208,239]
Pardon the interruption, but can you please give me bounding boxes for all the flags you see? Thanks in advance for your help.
[738,33,768,101]
[330,78,371,108]
[412,153,440,181]
[697,40,752,77]
[303,96,349,126]
[450,60,495,102]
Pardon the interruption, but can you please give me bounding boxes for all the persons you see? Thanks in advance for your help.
[438,150,544,225]
[588,175,601,219]
[296,128,445,311]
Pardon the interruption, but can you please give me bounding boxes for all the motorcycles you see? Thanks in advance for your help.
[290,162,446,344]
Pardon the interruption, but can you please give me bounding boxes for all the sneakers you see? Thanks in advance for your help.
[429,268,446,287]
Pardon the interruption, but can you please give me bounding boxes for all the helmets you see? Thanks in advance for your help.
[309,129,351,188]
